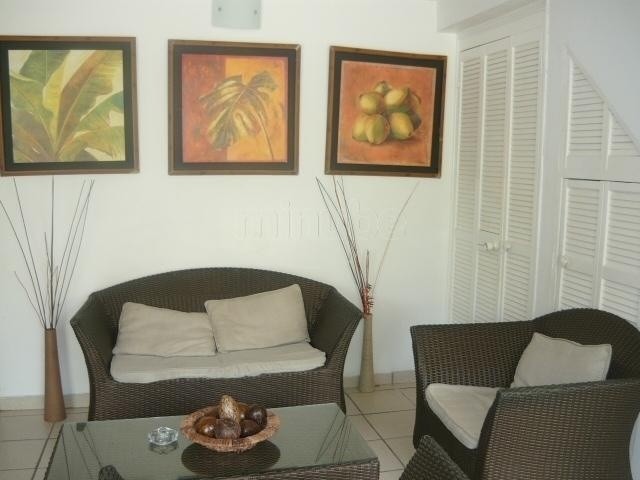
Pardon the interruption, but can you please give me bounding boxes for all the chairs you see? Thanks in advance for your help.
[410,303,639,479]
[96,434,468,480]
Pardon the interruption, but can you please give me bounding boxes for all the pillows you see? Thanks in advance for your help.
[112,299,216,359]
[512,330,613,391]
[205,284,311,353]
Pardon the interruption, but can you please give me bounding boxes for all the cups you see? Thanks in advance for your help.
[155,427,177,442]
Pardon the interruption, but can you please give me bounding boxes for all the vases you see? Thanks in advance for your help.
[357,311,377,394]
[42,327,67,423]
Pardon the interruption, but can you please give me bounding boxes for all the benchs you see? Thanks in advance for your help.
[69,265,366,422]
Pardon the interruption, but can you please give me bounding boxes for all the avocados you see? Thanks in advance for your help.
[194,394,267,439]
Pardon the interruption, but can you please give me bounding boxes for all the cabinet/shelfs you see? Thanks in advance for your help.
[451,33,544,323]
[555,48,639,328]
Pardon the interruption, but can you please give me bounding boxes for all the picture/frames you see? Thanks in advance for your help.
[323,44,447,179]
[166,36,302,177]
[0,36,138,177]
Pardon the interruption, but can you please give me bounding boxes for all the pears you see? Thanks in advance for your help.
[351,80,422,146]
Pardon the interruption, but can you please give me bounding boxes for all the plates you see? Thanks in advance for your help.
[148,428,178,445]
[181,402,278,452]
